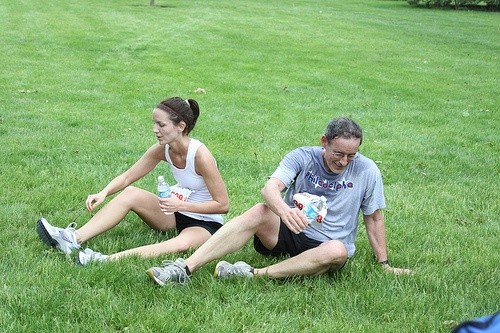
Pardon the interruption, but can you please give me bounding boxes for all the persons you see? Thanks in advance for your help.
[36,97,230,267]
[145,117,413,288]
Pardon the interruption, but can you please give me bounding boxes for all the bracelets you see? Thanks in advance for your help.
[377,260,388,266]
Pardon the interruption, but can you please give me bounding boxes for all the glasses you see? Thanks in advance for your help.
[330,147,359,160]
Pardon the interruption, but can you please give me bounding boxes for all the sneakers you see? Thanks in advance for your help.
[213,261,254,278]
[36,218,81,256]
[145,257,191,288]
[78,248,108,268]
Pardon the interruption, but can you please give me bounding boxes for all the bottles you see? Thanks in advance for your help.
[157,176,173,215]
[300,195,327,223]
